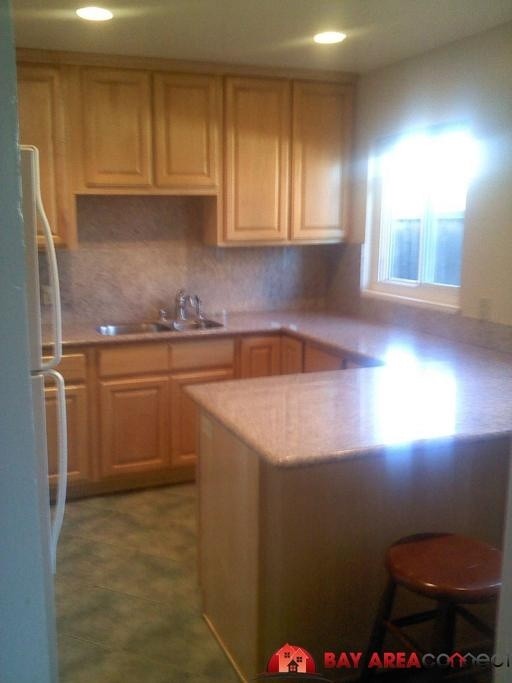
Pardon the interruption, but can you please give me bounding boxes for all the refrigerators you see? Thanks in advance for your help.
[0,0,71,683]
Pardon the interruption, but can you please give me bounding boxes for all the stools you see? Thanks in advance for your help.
[365,530,502,680]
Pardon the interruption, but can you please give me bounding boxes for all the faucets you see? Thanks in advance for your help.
[174,287,192,320]
[195,296,204,318]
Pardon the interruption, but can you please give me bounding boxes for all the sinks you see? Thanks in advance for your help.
[172,318,223,330]
[96,321,180,336]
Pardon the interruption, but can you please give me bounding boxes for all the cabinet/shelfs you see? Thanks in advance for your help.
[71,62,220,198]
[19,64,77,246]
[44,347,94,499]
[220,77,347,248]
[243,336,339,374]
[94,336,236,496]
